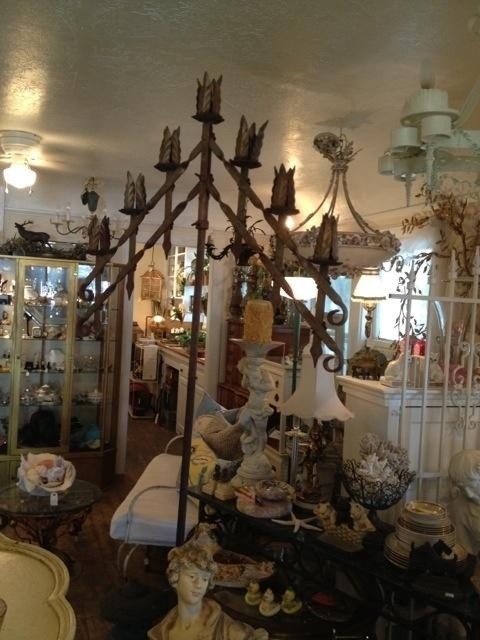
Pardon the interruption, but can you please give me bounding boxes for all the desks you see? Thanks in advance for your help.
[157,341,207,435]
[0,478,104,584]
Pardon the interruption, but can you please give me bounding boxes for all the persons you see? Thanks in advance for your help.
[447,449,480,555]
[146,539,270,640]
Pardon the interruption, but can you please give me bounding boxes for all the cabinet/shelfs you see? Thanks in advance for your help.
[334,373,480,526]
[166,245,197,322]
[0,252,128,491]
[182,487,479,637]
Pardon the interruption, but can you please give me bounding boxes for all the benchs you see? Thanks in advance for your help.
[108,433,201,590]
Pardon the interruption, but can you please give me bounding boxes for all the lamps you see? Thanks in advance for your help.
[280,349,356,510]
[372,9,478,208]
[266,120,402,275]
[347,269,394,350]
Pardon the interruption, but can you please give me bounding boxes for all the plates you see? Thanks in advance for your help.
[383,501,469,572]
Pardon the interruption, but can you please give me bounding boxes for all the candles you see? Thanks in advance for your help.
[241,298,275,343]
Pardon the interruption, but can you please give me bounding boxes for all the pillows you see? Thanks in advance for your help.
[174,391,244,517]
